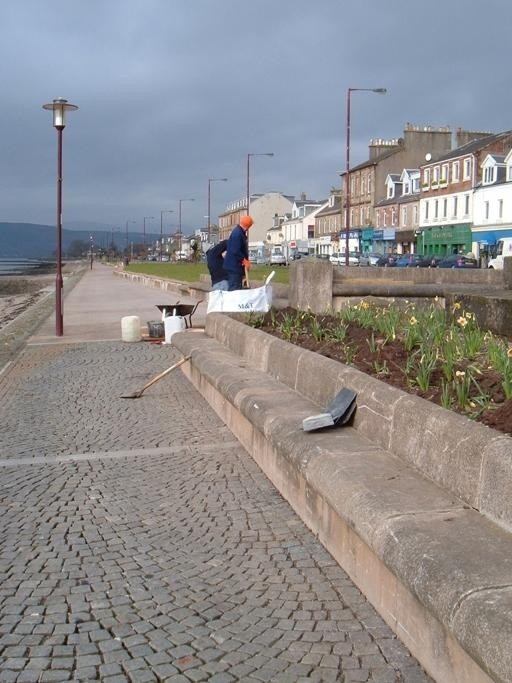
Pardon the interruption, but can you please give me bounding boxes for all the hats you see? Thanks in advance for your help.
[241,216,252,228]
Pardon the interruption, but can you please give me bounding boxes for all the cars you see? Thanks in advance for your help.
[430,254,478,269]
[350,251,364,267]
[139,248,187,263]
[397,253,425,268]
[288,252,310,265]
[358,252,382,267]
[201,253,207,261]
[314,254,329,259]
[329,252,346,265]
[249,251,257,263]
[425,255,444,267]
[376,252,402,267]
[268,253,287,267]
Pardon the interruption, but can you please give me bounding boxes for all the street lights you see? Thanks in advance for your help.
[416,229,425,256]
[247,152,274,255]
[125,220,137,259]
[42,96,81,337]
[160,210,174,262]
[207,178,228,243]
[345,86,389,270]
[99,227,120,254]
[89,236,94,269]
[177,197,197,262]
[143,216,155,261]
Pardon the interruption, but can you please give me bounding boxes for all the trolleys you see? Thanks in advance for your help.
[155,299,204,327]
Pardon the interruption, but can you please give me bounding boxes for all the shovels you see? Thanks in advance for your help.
[121,354,191,398]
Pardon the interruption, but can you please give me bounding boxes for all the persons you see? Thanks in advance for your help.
[202,239,229,291]
[467,250,475,258]
[223,216,253,291]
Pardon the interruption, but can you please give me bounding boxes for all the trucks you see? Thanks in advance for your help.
[483,236,512,270]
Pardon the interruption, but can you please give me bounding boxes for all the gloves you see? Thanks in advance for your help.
[242,258,249,269]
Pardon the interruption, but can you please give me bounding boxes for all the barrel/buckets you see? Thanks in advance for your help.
[121,316,140,343]
[147,321,164,338]
[165,316,186,344]
